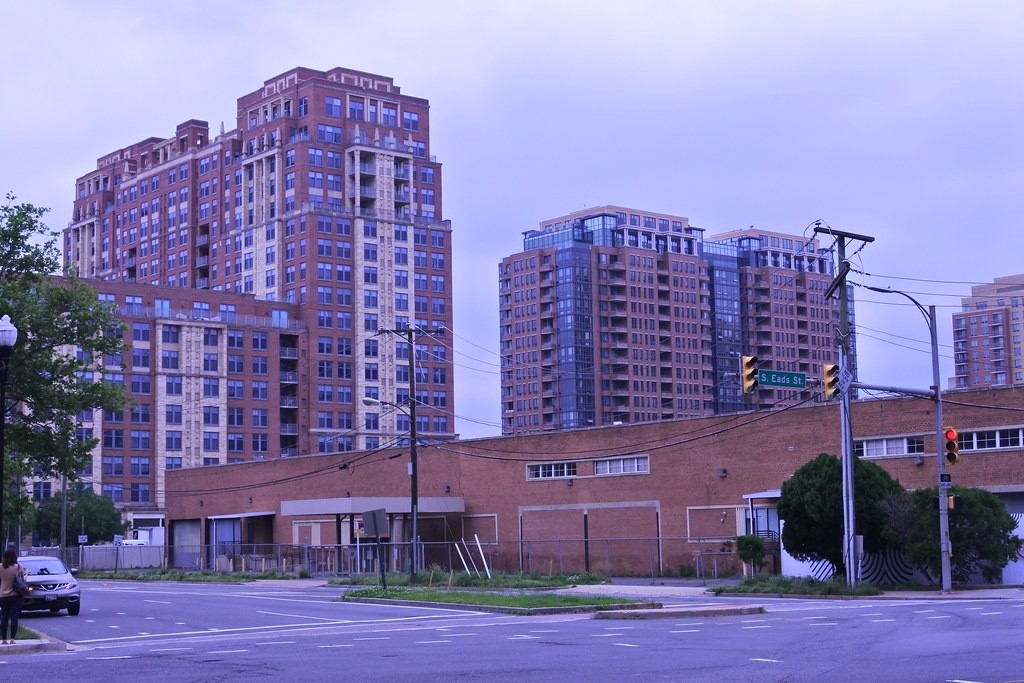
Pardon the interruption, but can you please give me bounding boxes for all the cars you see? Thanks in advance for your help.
[17,556,81,616]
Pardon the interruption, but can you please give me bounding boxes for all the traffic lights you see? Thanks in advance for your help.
[743,355,758,394]
[824,364,840,401]
[944,426,959,465]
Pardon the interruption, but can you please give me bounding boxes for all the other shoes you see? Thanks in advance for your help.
[2,642,8,644]
[10,641,16,644]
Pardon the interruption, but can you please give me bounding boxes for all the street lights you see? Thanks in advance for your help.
[866,285,951,594]
[0,313,18,560]
[361,398,418,585]
[38,504,43,548]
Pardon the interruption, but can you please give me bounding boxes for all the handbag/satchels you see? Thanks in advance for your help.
[13,564,30,594]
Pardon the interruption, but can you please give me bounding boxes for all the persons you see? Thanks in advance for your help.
[0,549,31,644]
